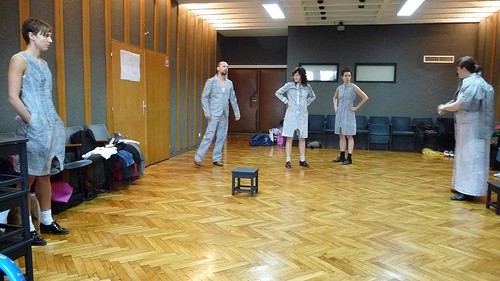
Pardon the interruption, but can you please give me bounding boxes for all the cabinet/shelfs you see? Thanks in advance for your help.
[0,135,34,281]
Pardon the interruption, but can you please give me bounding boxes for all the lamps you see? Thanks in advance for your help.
[337,21,345,31]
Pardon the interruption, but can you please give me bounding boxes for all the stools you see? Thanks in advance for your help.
[485,181,500,216]
[231,167,259,197]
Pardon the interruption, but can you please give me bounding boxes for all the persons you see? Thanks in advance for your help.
[275,67,316,168]
[332,68,369,165]
[437,56,494,201]
[7,18,69,245]
[194,61,241,166]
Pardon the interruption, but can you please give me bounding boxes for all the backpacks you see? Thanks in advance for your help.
[69,128,98,160]
[249,133,272,146]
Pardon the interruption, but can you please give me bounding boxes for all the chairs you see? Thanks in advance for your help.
[307,115,454,152]
[50,124,141,214]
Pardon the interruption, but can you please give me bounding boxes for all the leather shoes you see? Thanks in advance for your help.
[30,230,47,246]
[40,221,69,235]
[450,193,474,201]
[451,188,460,194]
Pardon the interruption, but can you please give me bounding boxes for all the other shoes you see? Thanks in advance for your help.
[213,161,223,166]
[300,161,309,167]
[342,159,352,164]
[194,159,201,166]
[333,157,345,162]
[286,161,291,168]
